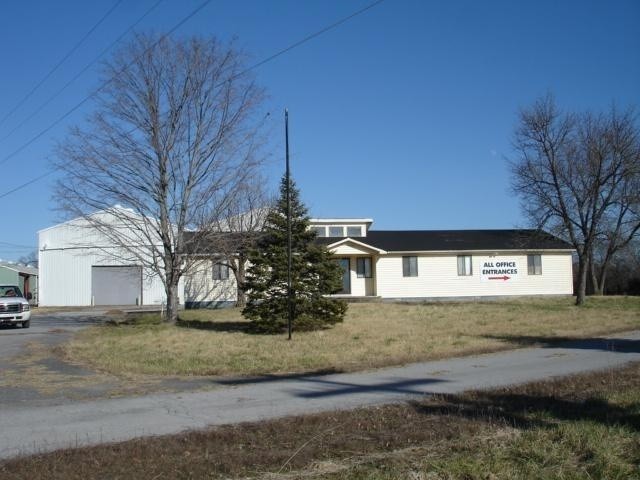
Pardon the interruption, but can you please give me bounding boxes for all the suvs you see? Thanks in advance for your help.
[0,283,34,328]
